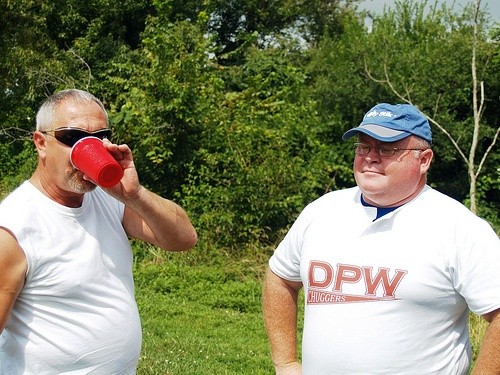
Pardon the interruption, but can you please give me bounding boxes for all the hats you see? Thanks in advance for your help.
[342,103,432,145]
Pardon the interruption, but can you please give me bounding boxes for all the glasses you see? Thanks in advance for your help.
[353,143,425,157]
[41,127,112,148]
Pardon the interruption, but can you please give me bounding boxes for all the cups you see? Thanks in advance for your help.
[70,136,123,188]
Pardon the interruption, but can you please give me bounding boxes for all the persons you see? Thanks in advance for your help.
[262,103,500,375]
[0,88,197,375]
